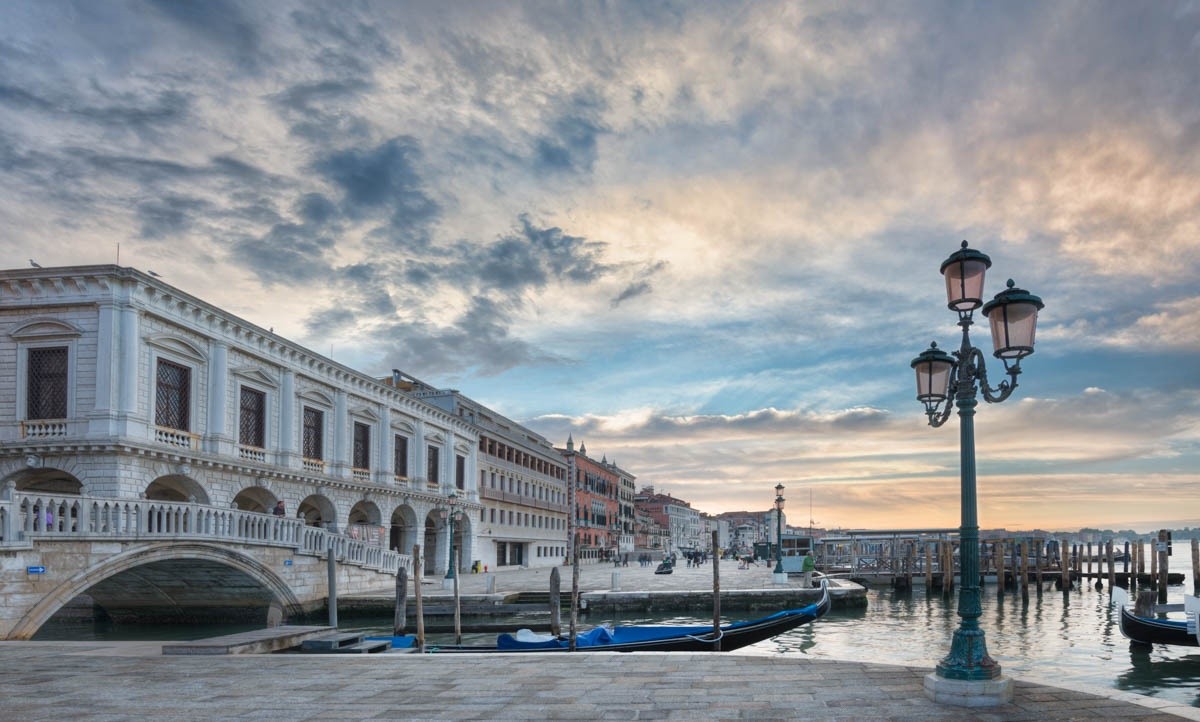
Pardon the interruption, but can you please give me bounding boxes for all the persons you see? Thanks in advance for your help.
[803,551,816,588]
[741,556,759,570]
[273,501,285,515]
[662,554,666,562]
[614,553,628,567]
[684,549,707,568]
[639,552,653,568]
[725,549,729,559]
[671,553,676,566]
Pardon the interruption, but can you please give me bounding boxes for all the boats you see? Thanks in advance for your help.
[363,579,831,651]
[1119,606,1200,648]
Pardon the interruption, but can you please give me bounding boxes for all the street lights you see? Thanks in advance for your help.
[774,483,786,573]
[616,526,621,556]
[439,492,465,579]
[912,236,1046,681]
